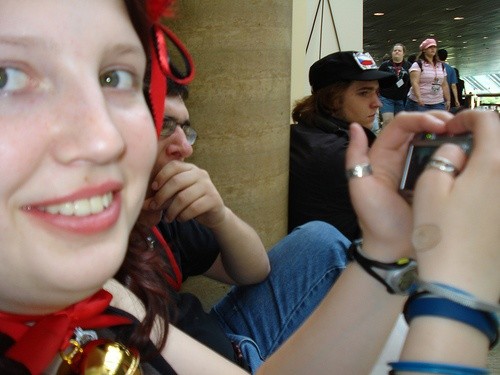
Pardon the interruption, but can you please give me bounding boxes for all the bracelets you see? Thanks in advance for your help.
[385,280,500,375]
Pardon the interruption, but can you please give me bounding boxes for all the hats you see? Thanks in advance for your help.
[419,39,437,50]
[309,50,394,88]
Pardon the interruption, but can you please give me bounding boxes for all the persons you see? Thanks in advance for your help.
[377,38,466,128]
[0,0,500,375]
[288,50,414,242]
[116,59,355,373]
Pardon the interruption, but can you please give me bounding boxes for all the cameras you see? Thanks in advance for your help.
[400,141,472,193]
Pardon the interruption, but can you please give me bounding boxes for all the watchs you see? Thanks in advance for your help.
[345,239,417,295]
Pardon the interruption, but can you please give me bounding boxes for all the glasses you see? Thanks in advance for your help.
[157,117,198,146]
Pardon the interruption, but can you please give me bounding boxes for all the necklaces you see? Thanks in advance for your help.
[135,216,181,292]
[0,289,143,375]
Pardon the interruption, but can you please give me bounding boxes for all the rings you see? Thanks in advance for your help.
[423,157,460,178]
[342,164,376,179]
